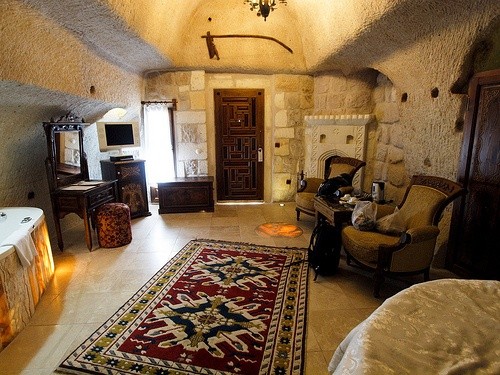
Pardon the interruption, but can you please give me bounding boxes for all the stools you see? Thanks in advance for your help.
[97,202,132,249]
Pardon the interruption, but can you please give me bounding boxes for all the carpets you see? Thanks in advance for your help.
[52,238,308,375]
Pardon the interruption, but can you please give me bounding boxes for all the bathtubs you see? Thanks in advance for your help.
[0,206,55,354]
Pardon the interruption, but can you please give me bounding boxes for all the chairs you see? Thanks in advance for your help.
[341,175,467,297]
[296,158,366,220]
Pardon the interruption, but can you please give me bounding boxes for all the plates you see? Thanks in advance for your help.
[340,197,350,200]
[347,201,357,205]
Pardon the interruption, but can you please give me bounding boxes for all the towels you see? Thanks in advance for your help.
[0,225,39,269]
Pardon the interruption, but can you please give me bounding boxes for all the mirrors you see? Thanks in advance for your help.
[55,130,83,180]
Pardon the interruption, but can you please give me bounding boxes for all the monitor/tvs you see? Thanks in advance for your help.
[96,121,140,156]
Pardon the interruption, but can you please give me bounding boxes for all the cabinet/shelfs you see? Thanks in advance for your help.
[101,158,151,219]
[41,110,119,252]
[158,175,215,215]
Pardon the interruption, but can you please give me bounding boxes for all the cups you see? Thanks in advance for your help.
[349,197,356,203]
[344,194,351,199]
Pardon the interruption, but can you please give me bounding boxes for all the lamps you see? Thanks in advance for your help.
[242,0,288,21]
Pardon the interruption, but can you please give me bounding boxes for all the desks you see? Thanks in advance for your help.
[314,195,385,228]
[328,278,500,375]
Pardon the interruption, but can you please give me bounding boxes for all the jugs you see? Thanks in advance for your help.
[371,182,384,202]
